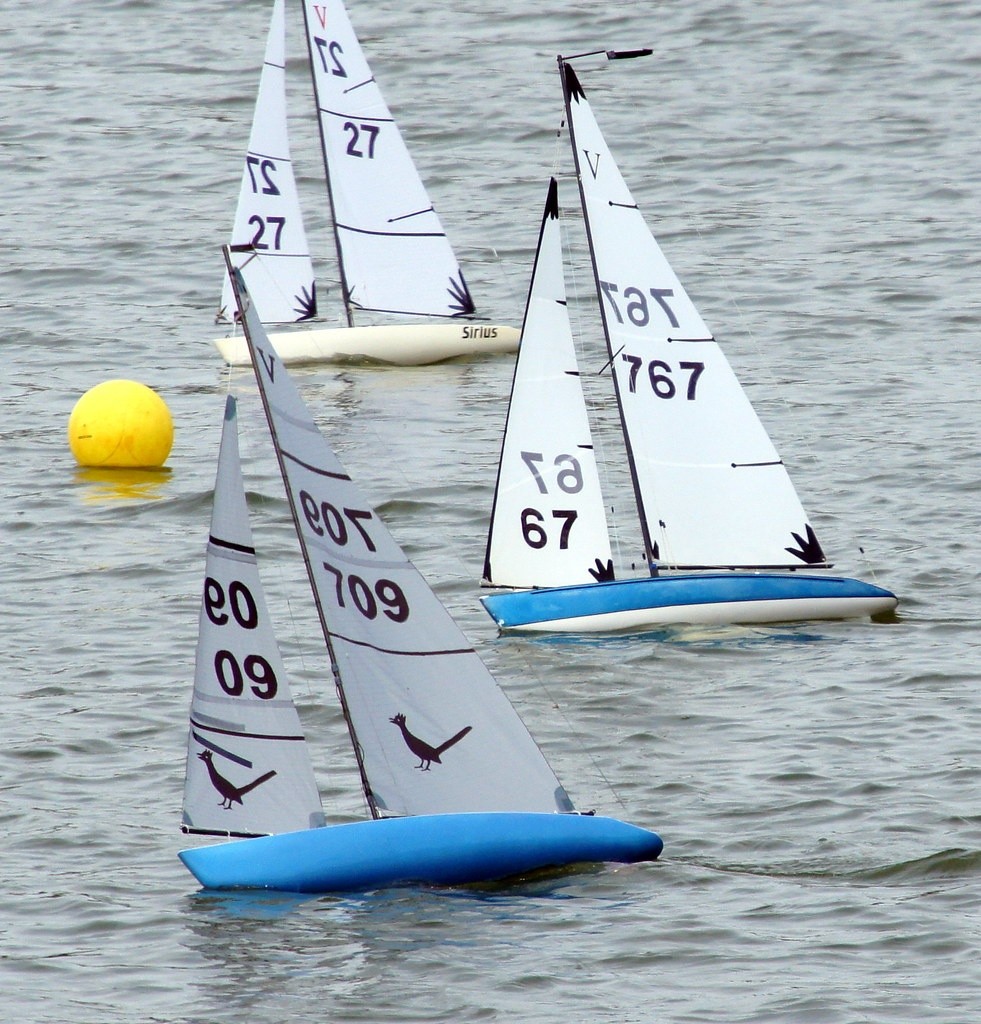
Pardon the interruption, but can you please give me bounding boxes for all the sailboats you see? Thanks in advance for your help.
[476,39,904,650]
[173,242,663,894]
[204,0,525,369]
